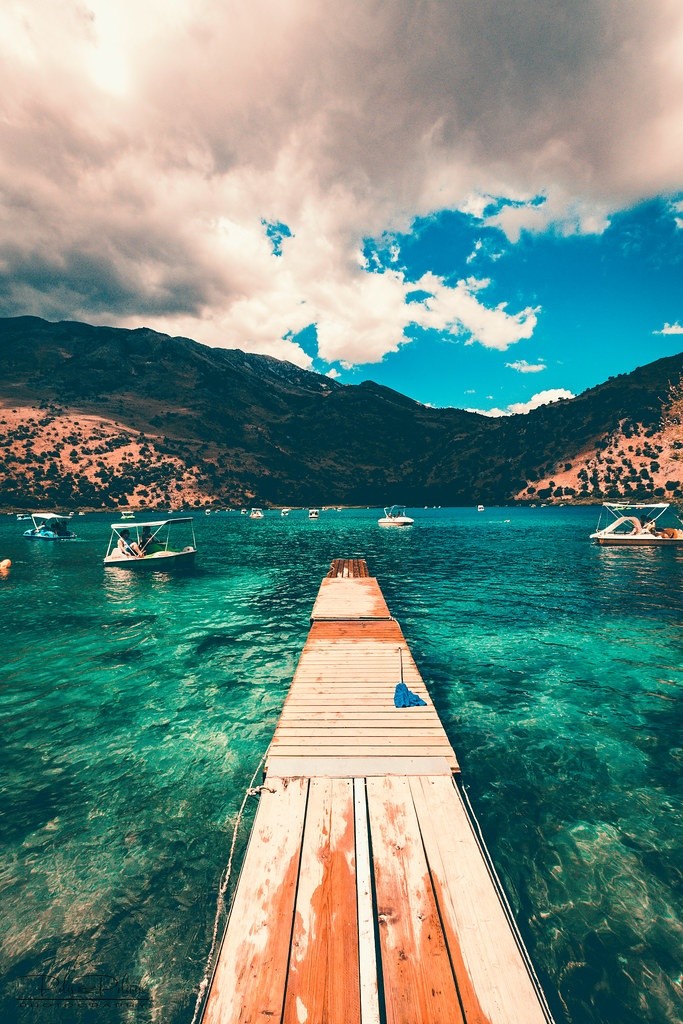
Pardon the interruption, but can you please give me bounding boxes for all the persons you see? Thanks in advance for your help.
[140,526,155,548]
[640,515,655,530]
[118,529,143,557]
[51,521,67,536]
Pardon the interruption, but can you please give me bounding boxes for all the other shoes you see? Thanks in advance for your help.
[670,532,674,538]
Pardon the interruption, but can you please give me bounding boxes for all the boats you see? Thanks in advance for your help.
[308,509,320,518]
[103,517,198,573]
[612,502,632,511]
[281,508,290,516]
[7,512,13,516]
[424,506,442,510]
[78,511,85,516]
[531,503,567,508]
[322,505,343,512]
[635,502,645,509]
[249,507,264,519]
[590,501,683,547]
[119,512,135,519]
[168,508,248,515]
[69,512,75,516]
[478,505,484,513]
[377,505,415,527]
[25,513,77,541]
[17,514,30,521]
